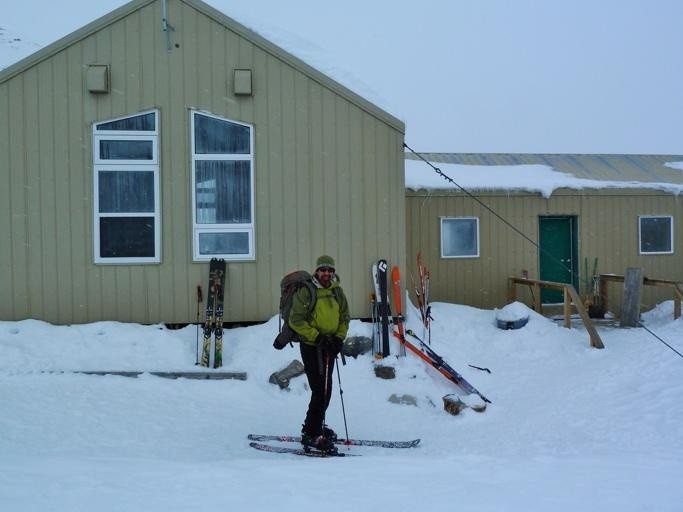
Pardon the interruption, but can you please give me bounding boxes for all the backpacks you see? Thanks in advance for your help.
[272,270,317,350]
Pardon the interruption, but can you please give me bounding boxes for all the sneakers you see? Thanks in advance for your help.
[300,423,337,442]
[300,432,334,450]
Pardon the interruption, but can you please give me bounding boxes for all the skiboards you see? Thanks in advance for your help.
[372,251,406,359]
[410,253,431,329]
[247,434,420,457]
[393,329,492,404]
[200,257,226,368]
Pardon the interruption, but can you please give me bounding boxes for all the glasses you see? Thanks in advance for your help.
[318,266,333,272]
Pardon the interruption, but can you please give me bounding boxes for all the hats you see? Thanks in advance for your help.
[315,255,335,271]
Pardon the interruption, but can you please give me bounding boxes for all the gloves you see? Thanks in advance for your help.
[313,333,342,355]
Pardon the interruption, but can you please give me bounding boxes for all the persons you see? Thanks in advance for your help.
[286,253,350,449]
